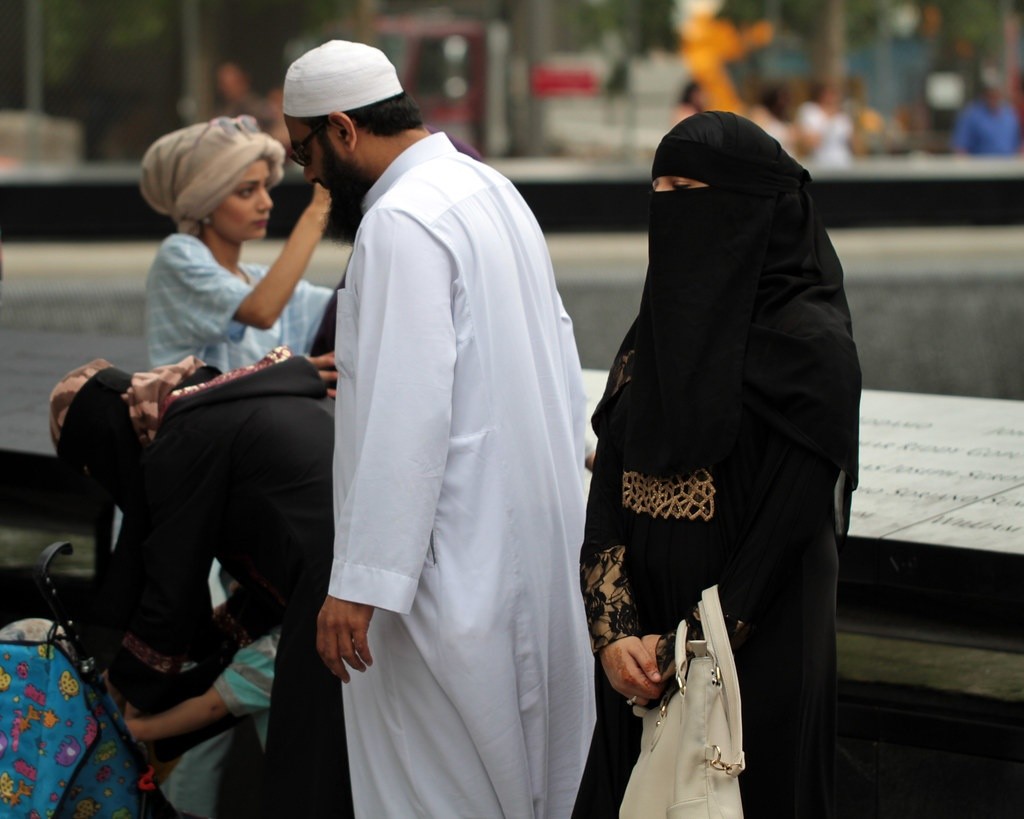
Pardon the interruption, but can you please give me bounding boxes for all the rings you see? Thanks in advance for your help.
[626,695,637,705]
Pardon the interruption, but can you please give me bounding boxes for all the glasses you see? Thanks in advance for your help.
[291,121,327,167]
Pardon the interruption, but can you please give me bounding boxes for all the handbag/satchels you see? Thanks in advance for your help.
[618,582,746,818]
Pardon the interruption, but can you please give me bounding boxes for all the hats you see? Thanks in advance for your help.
[282,39,405,119]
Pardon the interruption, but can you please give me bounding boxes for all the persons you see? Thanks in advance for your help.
[578,110,862,819]
[49,113,337,819]
[282,38,597,818]
[664,57,1020,172]
